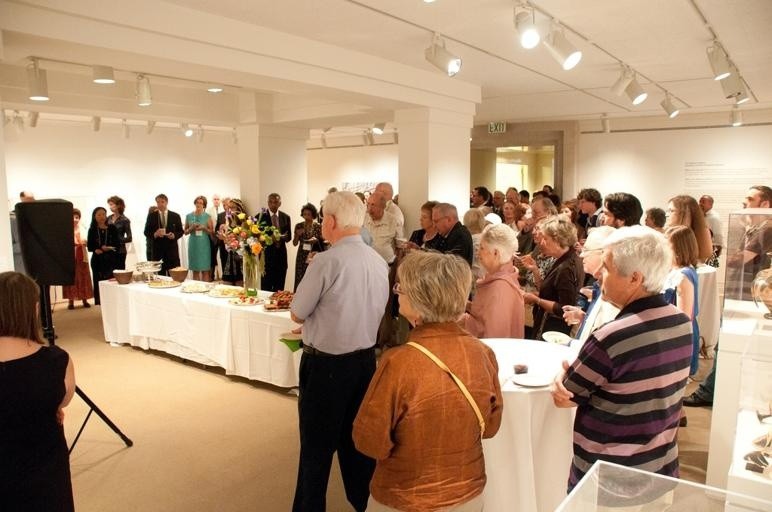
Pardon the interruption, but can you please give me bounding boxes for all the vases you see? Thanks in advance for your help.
[242,253,261,293]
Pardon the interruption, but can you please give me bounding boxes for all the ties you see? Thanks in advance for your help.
[161,211,165,228]
[272,214,278,231]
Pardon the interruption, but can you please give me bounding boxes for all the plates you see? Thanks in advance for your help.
[542,331,571,346]
[209,294,239,298]
[263,307,291,312]
[182,290,209,294]
[228,299,264,306]
[149,281,181,289]
[512,373,551,388]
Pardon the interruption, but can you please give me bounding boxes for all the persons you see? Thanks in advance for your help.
[0,271,76,512]
[508,204,532,238]
[457,223,525,339]
[254,194,292,293]
[471,186,494,210]
[665,225,699,428]
[207,195,224,280]
[523,214,579,341]
[559,225,621,350]
[665,196,709,269]
[62,208,93,310]
[293,202,327,293]
[645,208,667,235]
[502,200,515,225]
[217,199,250,286]
[107,195,132,270]
[506,187,519,206]
[575,193,642,312]
[576,188,606,246]
[392,201,444,319]
[87,207,120,305]
[514,216,558,340]
[680,186,772,408]
[518,185,562,212]
[184,196,214,282]
[517,196,558,255]
[144,194,185,277]
[353,251,504,512]
[404,203,473,272]
[699,195,723,258]
[20,191,35,203]
[559,201,576,220]
[361,192,404,287]
[548,224,693,512]
[290,193,390,512]
[215,198,232,278]
[492,191,504,223]
[146,206,159,262]
[318,183,405,225]
[464,210,486,278]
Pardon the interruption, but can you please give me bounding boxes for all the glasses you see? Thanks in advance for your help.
[393,282,407,298]
[579,247,598,255]
[432,219,442,225]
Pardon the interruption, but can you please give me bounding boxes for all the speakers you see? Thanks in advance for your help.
[14,199,76,288]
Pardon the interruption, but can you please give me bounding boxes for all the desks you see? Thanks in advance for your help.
[98,274,304,389]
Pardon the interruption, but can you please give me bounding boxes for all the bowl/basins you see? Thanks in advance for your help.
[169,271,189,282]
[113,271,133,284]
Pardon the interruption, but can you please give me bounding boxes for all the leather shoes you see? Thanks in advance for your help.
[83,301,92,308]
[68,304,75,310]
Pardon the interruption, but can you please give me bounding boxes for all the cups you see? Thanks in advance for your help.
[564,306,581,325]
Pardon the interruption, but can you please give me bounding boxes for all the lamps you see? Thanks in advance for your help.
[144,121,155,137]
[543,20,580,70]
[373,124,386,135]
[2,109,11,129]
[721,67,743,100]
[718,61,719,62]
[91,65,116,84]
[625,80,647,105]
[195,125,204,145]
[367,128,373,145]
[23,56,49,101]
[610,68,635,96]
[92,116,101,132]
[362,130,368,145]
[660,91,679,119]
[27,111,39,128]
[11,115,24,132]
[513,3,540,50]
[705,42,730,81]
[732,110,742,127]
[425,31,462,78]
[179,122,193,137]
[736,88,750,105]
[121,120,129,138]
[134,73,152,107]
[600,116,610,134]
[320,134,327,148]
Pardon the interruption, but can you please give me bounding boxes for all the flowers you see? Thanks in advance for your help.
[216,207,282,291]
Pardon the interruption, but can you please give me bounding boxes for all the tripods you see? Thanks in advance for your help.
[23,286,134,462]
